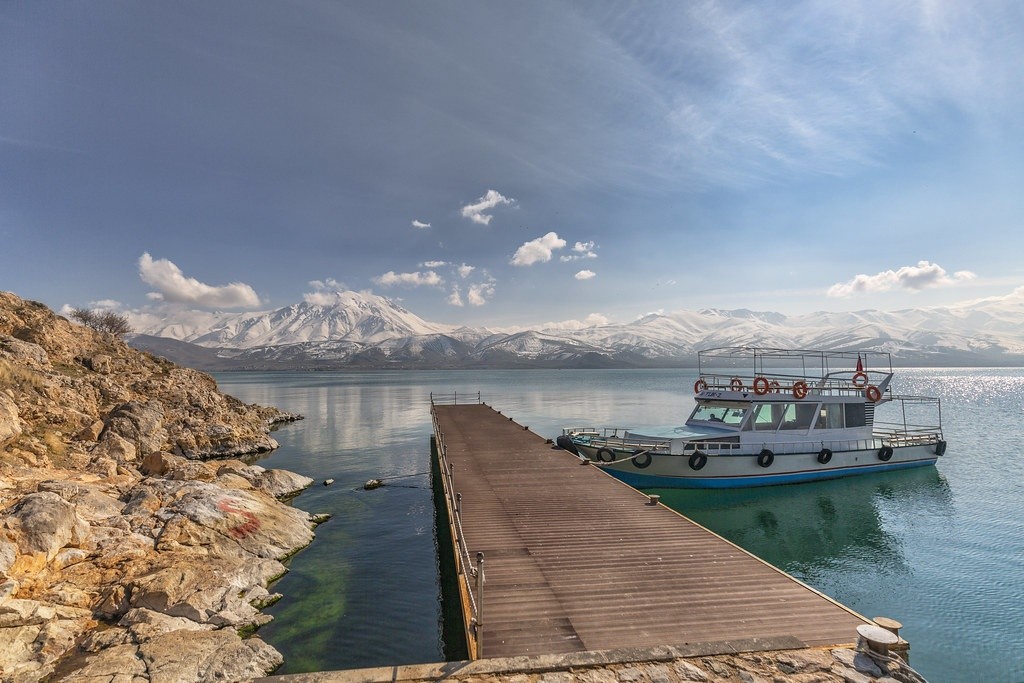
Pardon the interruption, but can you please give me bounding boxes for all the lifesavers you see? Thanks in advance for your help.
[818,449,834,464]
[688,450,708,472]
[754,377,769,395]
[694,380,708,394]
[935,440,947,456]
[769,381,780,394]
[853,373,869,388]
[596,446,616,467]
[867,386,882,402]
[756,449,775,468]
[878,445,894,462]
[631,448,653,469]
[730,379,742,391]
[792,382,808,400]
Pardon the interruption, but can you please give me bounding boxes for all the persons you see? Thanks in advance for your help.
[708,414,724,423]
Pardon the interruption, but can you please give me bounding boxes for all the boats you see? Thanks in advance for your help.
[556,344,947,490]
